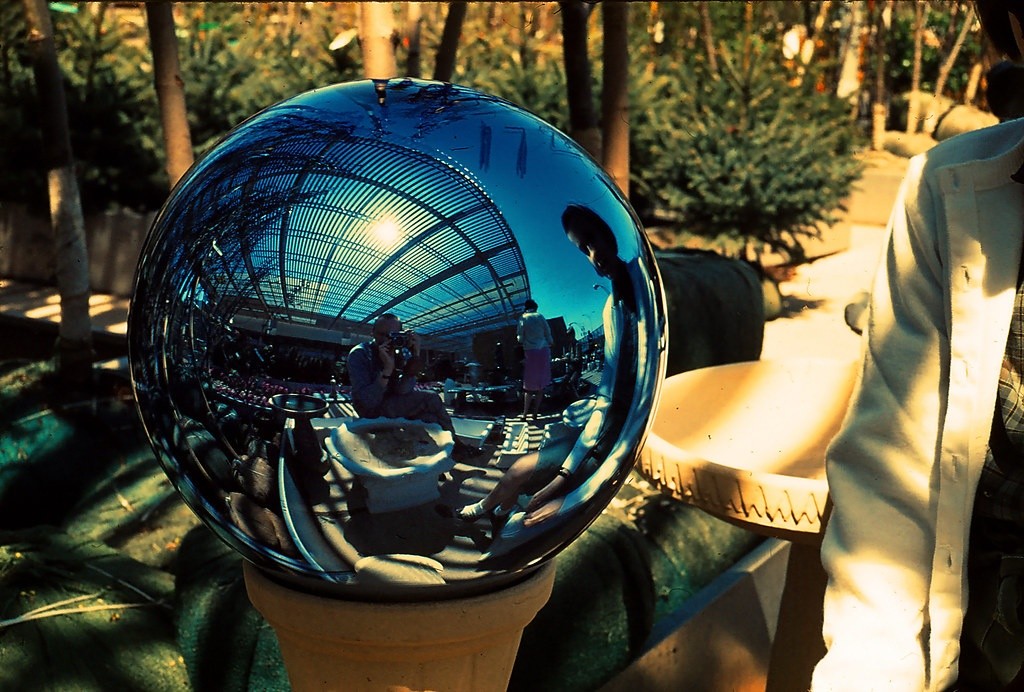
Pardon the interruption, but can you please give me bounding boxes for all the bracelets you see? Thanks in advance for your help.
[379,371,391,379]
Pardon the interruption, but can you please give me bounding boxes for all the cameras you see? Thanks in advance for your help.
[388,330,414,348]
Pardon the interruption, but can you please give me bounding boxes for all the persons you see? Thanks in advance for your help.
[809,0,1024,692]
[453,204,658,562]
[346,314,486,461]
[516,300,553,422]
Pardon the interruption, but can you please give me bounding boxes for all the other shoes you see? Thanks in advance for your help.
[521,416,527,422]
[482,503,510,522]
[532,415,537,420]
[434,499,480,522]
[453,441,480,458]
